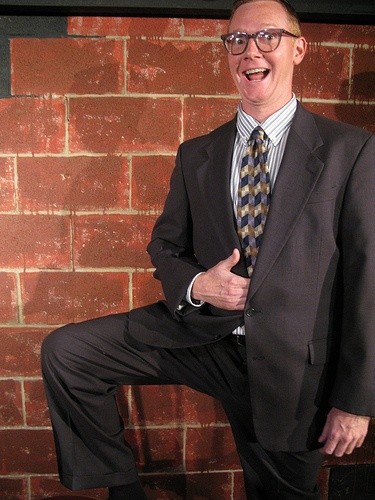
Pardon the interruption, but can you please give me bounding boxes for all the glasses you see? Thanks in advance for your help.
[220,28,300,56]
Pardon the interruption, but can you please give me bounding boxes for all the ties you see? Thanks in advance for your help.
[235,125,273,277]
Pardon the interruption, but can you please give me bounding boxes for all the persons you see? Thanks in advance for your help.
[41,0,375,500]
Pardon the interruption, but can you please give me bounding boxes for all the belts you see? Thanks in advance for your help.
[225,333,246,348]
